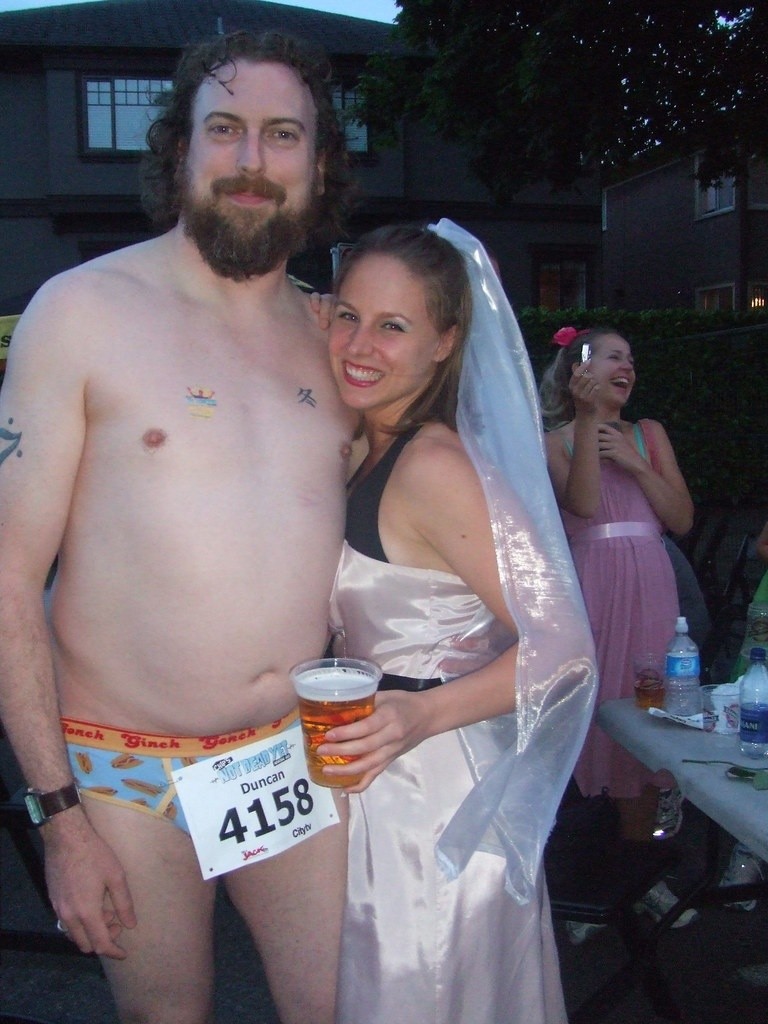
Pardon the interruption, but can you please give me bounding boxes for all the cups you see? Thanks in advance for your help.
[290,657,383,788]
[633,651,665,709]
[700,683,740,750]
[740,602,768,659]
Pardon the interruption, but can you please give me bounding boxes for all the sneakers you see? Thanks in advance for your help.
[719,842,764,911]
[632,880,701,928]
[651,788,684,841]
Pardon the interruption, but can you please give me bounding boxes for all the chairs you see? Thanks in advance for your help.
[543,775,687,1024]
[677,510,757,686]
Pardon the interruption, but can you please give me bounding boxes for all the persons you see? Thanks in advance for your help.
[0,31,696,1024]
[756,519,768,557]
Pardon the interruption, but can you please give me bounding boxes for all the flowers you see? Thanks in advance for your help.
[553,327,590,347]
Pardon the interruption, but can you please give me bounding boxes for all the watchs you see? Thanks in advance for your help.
[22,778,81,827]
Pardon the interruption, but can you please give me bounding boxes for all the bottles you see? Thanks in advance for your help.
[736,648,768,759]
[663,617,702,716]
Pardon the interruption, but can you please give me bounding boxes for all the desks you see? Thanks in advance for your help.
[595,698,768,1024]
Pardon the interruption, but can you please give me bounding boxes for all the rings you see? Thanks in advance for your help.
[56,918,70,934]
